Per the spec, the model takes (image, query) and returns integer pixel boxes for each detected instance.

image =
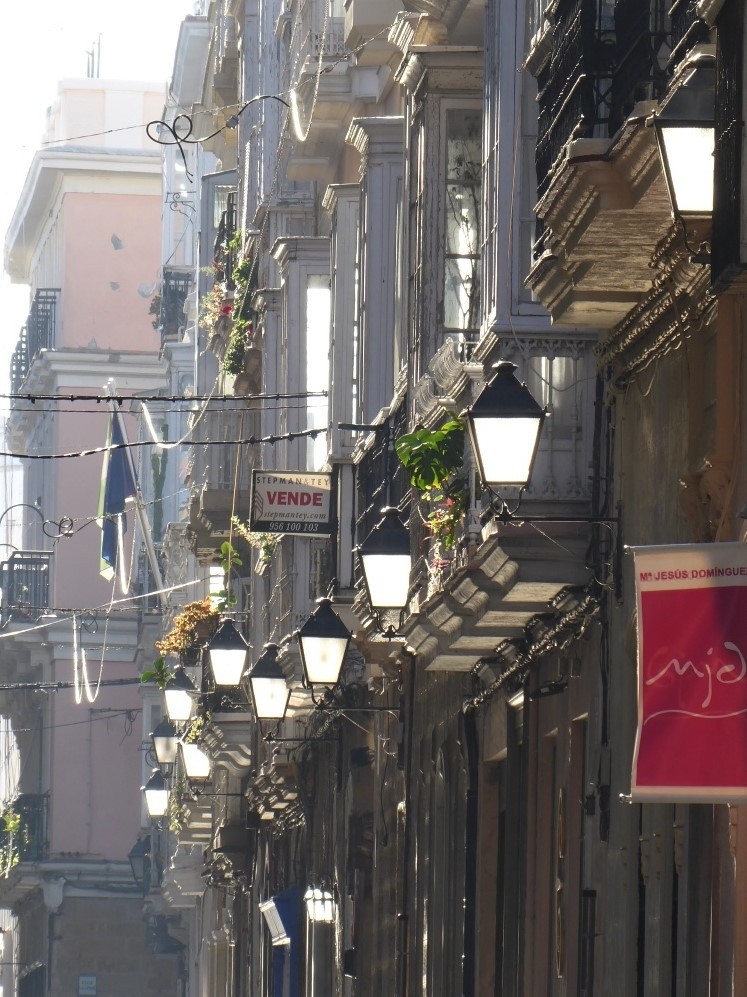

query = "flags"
[96,403,140,581]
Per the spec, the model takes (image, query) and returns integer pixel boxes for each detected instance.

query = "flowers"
[156,596,220,657]
[395,411,472,573]
[196,257,257,375]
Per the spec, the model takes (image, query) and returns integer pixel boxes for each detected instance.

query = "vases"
[180,621,210,667]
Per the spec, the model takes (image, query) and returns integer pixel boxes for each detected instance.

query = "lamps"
[645,54,715,269]
[303,881,339,924]
[243,642,340,742]
[459,362,624,522]
[356,507,411,637]
[179,726,245,796]
[126,834,150,895]
[159,665,197,722]
[296,598,401,711]
[140,715,179,816]
[207,618,252,705]
